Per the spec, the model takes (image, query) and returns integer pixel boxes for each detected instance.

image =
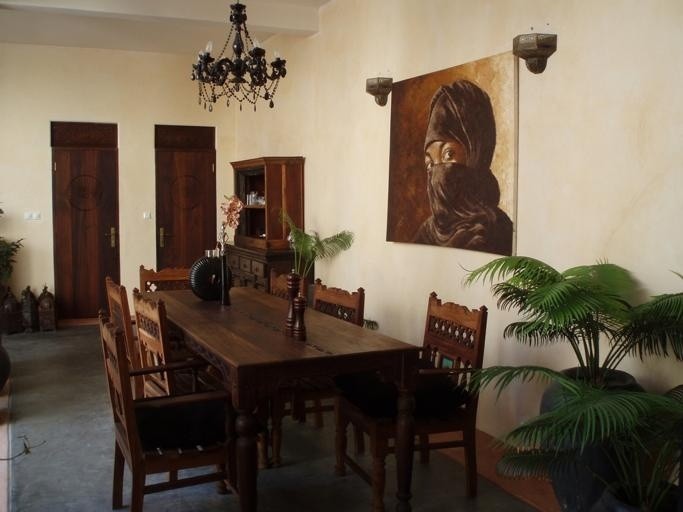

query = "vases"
[219,224,229,305]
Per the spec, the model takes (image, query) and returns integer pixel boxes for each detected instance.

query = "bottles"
[284,273,306,335]
[189,249,232,301]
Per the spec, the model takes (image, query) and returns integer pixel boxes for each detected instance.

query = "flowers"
[219,193,244,231]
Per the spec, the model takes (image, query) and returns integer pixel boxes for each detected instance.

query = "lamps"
[366,78,393,106]
[190,0,286,111]
[513,33,557,73]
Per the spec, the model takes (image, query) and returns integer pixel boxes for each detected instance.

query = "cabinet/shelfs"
[226,157,315,297]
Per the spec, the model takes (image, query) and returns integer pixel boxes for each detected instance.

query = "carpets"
[1,325,541,511]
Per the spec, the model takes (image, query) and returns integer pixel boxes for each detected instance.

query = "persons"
[413,78,511,257]
